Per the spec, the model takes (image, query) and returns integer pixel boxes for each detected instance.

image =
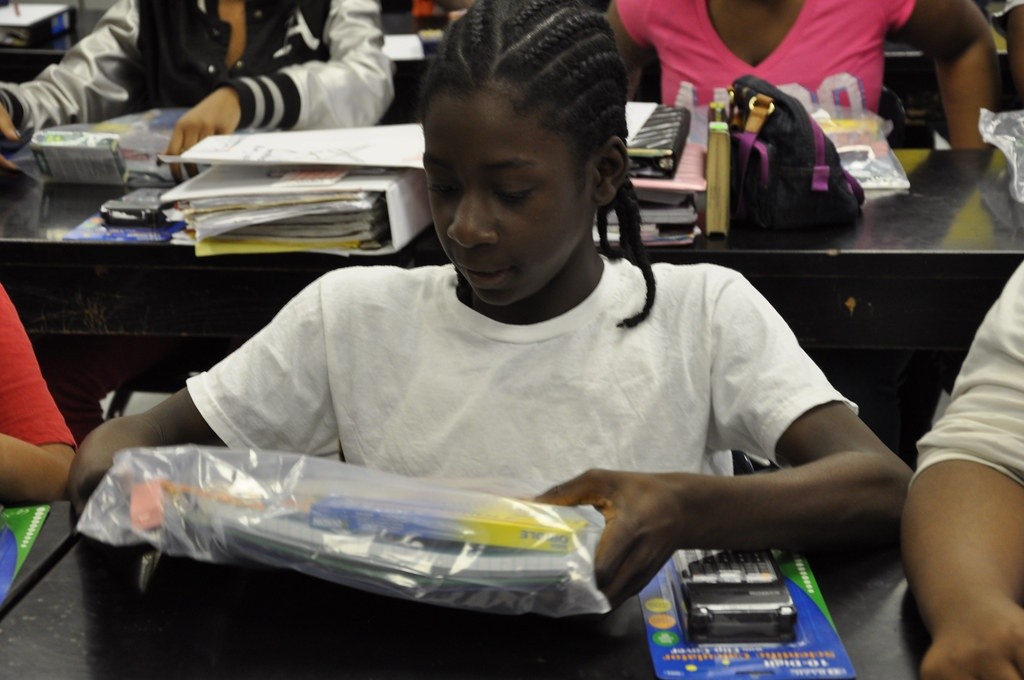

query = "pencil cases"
[624,102,691,181]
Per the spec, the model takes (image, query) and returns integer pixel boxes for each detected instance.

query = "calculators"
[674,547,799,645]
[98,185,170,226]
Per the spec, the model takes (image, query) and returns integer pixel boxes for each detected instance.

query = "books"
[593,189,703,246]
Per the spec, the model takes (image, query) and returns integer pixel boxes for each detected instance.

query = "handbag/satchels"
[710,74,864,240]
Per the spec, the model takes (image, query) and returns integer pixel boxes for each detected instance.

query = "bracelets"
[79,468,108,502]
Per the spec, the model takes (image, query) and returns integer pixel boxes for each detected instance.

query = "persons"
[591,0,998,158]
[0,283,77,504]
[1,0,397,180]
[993,1,1024,106]
[902,262,1023,680]
[70,1,917,614]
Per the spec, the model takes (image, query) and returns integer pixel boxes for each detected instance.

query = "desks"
[0,16,1024,680]
